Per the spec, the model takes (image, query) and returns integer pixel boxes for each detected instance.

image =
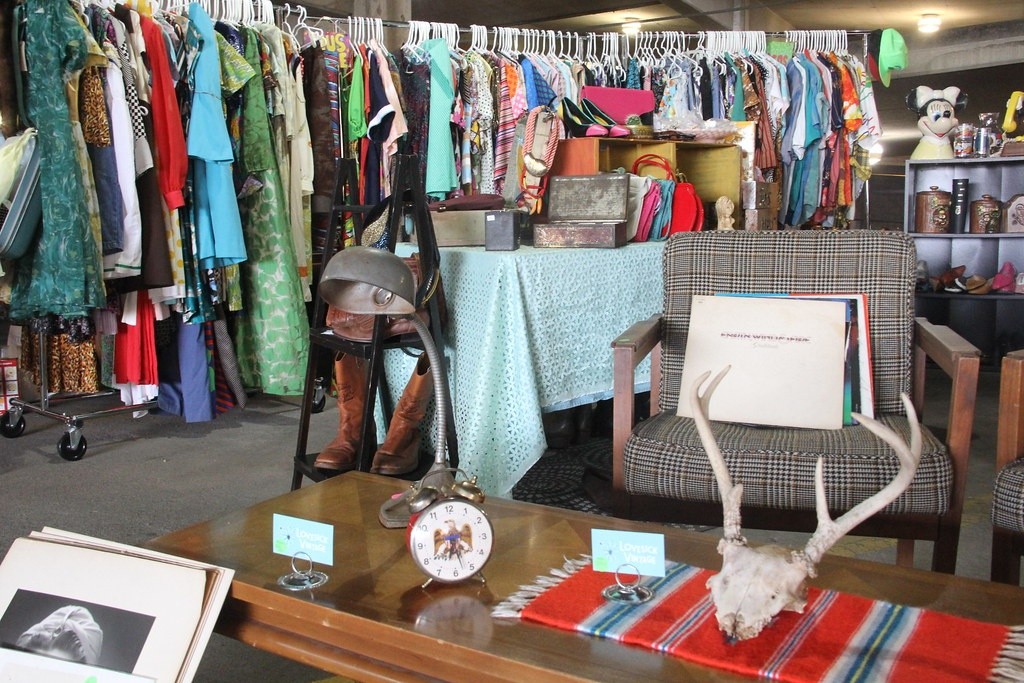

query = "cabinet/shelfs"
[901,156,1023,374]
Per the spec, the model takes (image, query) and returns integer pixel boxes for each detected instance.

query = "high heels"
[557,97,633,140]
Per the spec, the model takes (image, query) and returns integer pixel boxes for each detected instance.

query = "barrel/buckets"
[914,186,951,233]
[970,195,1003,233]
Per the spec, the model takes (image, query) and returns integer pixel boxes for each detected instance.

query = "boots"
[312,349,378,469]
[372,352,434,474]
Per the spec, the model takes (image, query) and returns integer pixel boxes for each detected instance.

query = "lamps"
[318,245,469,529]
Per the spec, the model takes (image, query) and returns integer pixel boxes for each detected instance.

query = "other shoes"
[919,261,1023,296]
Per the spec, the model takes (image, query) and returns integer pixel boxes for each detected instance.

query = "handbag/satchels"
[619,153,706,243]
[327,249,448,342]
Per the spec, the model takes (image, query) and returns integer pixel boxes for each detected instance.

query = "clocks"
[403,466,494,589]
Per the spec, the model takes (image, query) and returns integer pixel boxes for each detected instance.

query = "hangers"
[78,0,881,86]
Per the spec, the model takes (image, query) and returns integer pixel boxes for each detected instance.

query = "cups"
[973,126,991,158]
[951,178,969,233]
[953,122,977,158]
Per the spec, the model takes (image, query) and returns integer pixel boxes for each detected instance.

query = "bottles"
[974,112,1005,153]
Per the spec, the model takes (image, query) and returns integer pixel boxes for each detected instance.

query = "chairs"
[608,228,984,579]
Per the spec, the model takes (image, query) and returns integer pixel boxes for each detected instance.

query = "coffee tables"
[128,466,1023,683]
